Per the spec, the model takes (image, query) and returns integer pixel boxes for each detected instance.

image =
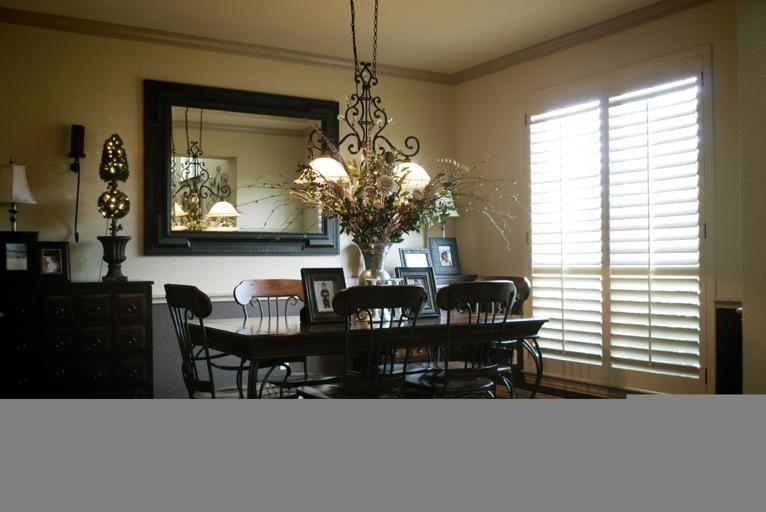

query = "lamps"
[64,123,87,243]
[294,0,432,203]
[169,106,245,232]
[95,132,132,282]
[1,156,39,231]
[427,183,462,237]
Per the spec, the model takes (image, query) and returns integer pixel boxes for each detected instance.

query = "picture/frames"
[426,234,464,276]
[396,246,434,279]
[39,239,72,281]
[300,266,354,323]
[0,229,39,276]
[392,264,441,319]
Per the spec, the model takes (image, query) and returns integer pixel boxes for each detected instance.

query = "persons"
[412,278,425,289]
[45,254,62,274]
[320,283,331,309]
[440,249,452,266]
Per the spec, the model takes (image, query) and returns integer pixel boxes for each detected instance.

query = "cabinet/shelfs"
[1,271,156,399]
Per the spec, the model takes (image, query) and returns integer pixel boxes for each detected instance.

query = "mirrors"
[140,76,344,257]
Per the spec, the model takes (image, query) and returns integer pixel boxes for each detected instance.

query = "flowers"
[236,119,532,252]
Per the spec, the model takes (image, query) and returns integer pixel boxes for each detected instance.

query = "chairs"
[163,283,301,399]
[473,273,532,390]
[234,277,339,388]
[400,280,518,399]
[294,284,428,402]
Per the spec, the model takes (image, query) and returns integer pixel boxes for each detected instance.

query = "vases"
[348,236,399,321]
